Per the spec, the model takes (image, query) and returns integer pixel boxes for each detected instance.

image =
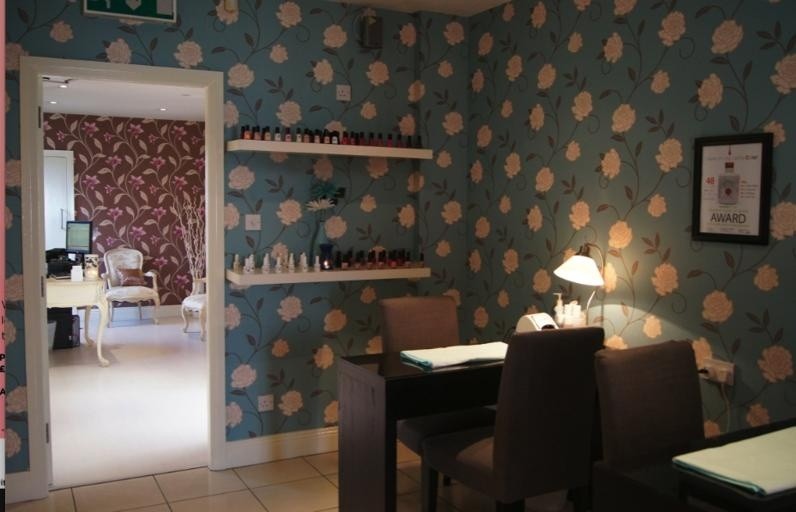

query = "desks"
[46,277,110,367]
[667,417,796,510]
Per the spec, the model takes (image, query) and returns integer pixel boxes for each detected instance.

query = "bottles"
[240,124,424,152]
[234,243,422,273]
[71,254,98,280]
[563,303,587,330]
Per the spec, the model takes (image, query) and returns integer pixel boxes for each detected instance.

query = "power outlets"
[335,84,352,102]
[255,395,275,414]
[700,358,734,387]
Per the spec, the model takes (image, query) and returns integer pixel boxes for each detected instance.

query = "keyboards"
[49,268,71,280]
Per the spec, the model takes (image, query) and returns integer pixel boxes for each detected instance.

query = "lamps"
[553,243,605,326]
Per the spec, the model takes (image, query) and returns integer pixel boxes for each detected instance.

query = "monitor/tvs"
[65,221,93,262]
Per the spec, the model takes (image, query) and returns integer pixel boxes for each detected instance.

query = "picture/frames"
[692,133,774,247]
[82,0,177,24]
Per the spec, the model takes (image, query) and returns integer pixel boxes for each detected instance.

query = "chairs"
[100,249,161,329]
[594,340,705,511]
[181,276,207,343]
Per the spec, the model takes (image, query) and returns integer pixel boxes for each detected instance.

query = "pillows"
[115,268,144,286]
